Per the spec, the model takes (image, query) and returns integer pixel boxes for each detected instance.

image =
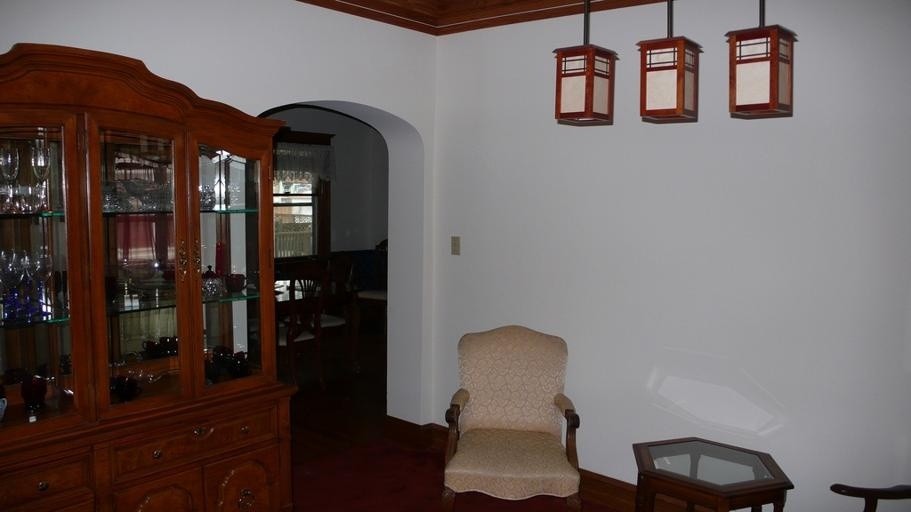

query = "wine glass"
[0,147,56,319]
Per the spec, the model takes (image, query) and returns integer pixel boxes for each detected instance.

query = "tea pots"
[201,264,222,299]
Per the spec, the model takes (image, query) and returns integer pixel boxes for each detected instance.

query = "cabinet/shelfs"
[85,109,276,420]
[98,382,299,512]
[0,421,106,512]
[0,107,97,450]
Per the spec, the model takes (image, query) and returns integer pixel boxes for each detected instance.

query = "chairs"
[441,324,585,511]
[829,484,911,512]
[275,253,352,394]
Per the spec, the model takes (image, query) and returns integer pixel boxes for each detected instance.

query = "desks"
[353,289,387,387]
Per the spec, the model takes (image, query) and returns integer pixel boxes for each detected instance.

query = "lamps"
[552,0,620,126]
[724,0,799,119]
[635,0,703,121]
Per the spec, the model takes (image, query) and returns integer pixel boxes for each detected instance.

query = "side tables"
[632,435,795,512]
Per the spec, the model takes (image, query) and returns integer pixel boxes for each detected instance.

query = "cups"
[141,335,176,357]
[20,377,47,413]
[226,274,248,292]
